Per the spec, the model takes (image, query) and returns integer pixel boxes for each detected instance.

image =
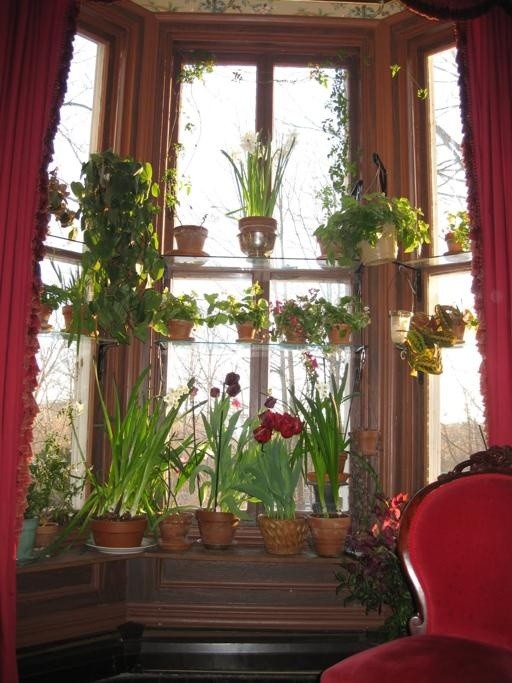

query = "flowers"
[221,128,299,216]
[161,44,214,225]
[332,490,419,639]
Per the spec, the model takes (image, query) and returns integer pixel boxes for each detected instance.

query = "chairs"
[320,444,512,683]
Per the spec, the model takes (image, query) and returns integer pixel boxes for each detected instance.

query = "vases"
[173,225,209,251]
[236,216,279,257]
[348,430,380,456]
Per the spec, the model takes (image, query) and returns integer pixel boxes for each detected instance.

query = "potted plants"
[314,190,471,266]
